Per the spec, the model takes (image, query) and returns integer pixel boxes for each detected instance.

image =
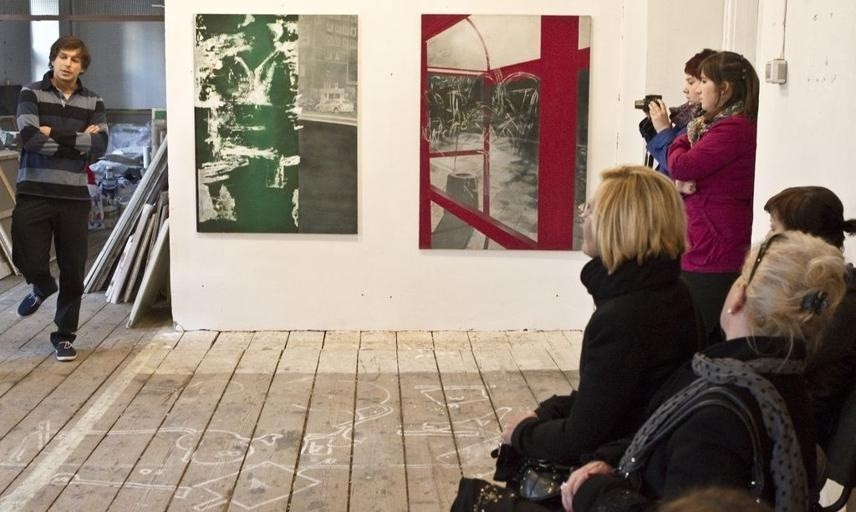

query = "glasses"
[749,233,787,283]
[577,203,593,219]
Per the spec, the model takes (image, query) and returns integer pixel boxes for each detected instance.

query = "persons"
[666,52,760,343]
[763,185,855,512]
[448,228,850,511]
[645,49,721,177]
[9,35,110,362]
[499,163,706,467]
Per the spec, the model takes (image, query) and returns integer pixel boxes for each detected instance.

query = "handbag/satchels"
[520,458,577,501]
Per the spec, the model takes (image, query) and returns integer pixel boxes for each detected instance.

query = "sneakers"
[16,288,58,318]
[54,340,77,361]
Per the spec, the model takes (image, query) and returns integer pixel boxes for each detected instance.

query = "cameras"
[635,94,663,114]
